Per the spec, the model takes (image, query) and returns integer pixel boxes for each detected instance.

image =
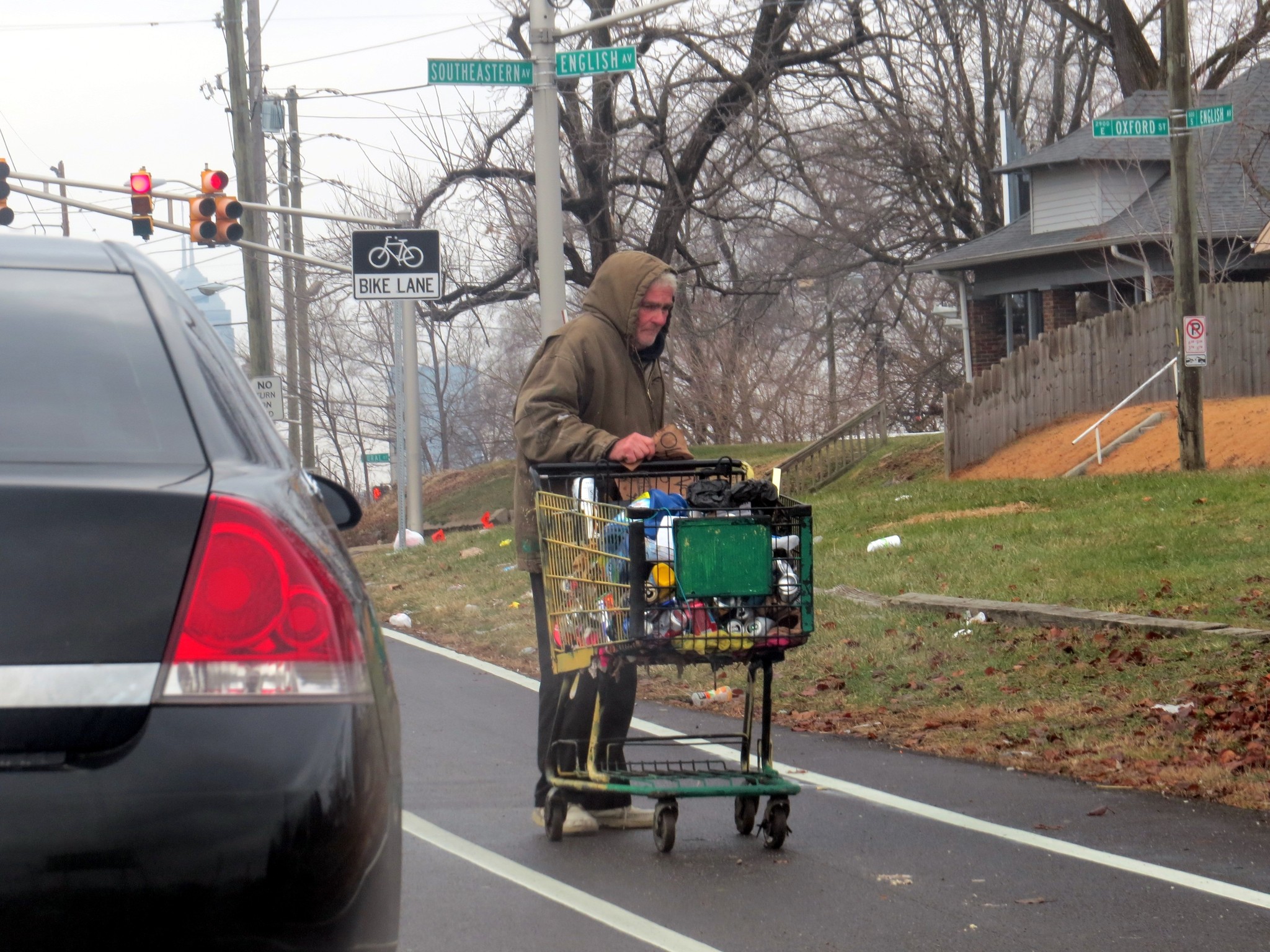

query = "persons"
[512,251,677,832]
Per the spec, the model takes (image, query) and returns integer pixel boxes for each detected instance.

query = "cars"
[0,230,408,952]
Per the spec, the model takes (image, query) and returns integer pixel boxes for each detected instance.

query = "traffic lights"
[0,158,15,227]
[215,195,244,244]
[201,170,229,194]
[129,171,154,236]
[189,195,218,244]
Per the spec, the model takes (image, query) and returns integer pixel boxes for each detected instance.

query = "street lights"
[196,281,300,464]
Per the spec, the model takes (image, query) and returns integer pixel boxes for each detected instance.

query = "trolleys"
[527,457,819,854]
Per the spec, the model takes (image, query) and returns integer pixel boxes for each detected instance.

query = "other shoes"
[532,802,598,833]
[587,804,656,830]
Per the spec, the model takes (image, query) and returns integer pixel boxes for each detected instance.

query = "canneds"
[571,476,601,540]
[552,555,801,649]
